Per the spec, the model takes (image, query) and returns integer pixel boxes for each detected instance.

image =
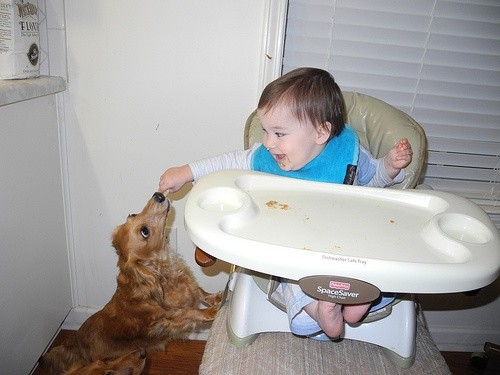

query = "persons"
[160,67,414,339]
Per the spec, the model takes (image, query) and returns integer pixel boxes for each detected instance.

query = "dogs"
[38,192,225,375]
[61,346,147,375]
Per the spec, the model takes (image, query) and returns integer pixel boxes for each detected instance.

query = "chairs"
[193,88,456,375]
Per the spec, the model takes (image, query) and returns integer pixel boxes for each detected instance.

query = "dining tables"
[181,167,500,369]
